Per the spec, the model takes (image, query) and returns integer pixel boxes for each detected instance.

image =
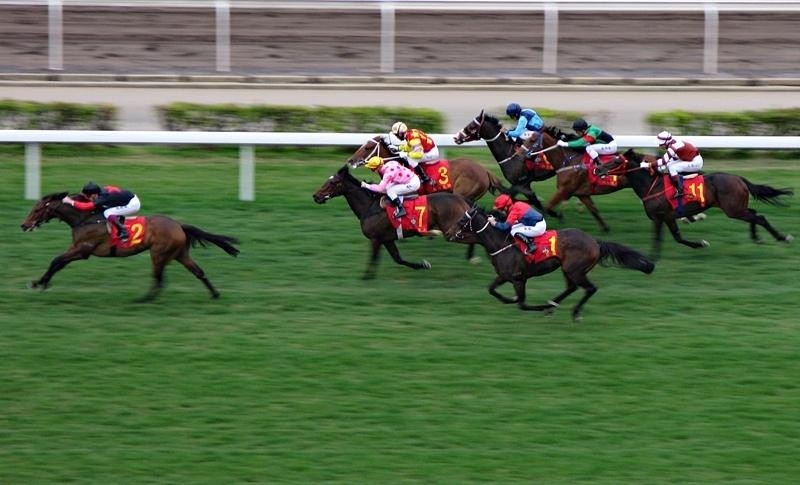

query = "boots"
[113,218,129,239]
[414,164,431,184]
[670,174,684,199]
[593,156,602,166]
[514,232,537,253]
[392,197,407,218]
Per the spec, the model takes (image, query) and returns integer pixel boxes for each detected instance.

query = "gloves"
[639,161,649,168]
[360,181,371,189]
[388,144,399,150]
[396,151,408,158]
[61,196,74,206]
[557,139,568,147]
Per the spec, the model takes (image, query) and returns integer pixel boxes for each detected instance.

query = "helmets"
[570,118,588,130]
[364,156,383,169]
[391,122,408,135]
[656,131,673,144]
[82,182,101,195]
[506,102,521,119]
[494,193,512,208]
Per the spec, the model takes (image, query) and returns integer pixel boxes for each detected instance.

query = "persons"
[63,182,141,240]
[557,119,617,166]
[361,156,421,218]
[388,121,439,184]
[487,194,546,256]
[500,103,544,154]
[640,130,703,199]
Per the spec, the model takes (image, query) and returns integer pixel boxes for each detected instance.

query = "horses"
[445,203,655,323]
[346,136,509,202]
[515,124,707,233]
[20,191,241,304]
[312,163,483,280]
[452,108,565,221]
[595,147,795,259]
